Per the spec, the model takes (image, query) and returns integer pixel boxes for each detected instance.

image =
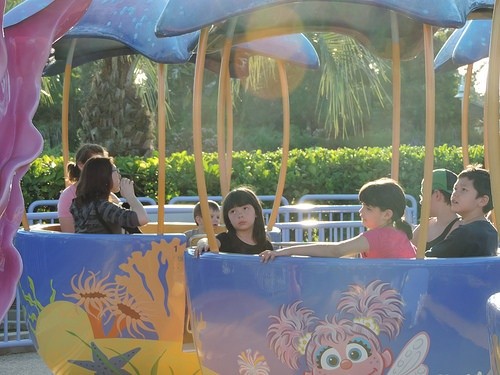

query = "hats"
[421,169,458,195]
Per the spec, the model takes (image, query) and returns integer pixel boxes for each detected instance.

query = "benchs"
[13,222,500,375]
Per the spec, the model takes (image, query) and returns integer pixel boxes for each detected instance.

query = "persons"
[410,168,500,258]
[184,188,273,258]
[259,179,418,264]
[58,143,149,234]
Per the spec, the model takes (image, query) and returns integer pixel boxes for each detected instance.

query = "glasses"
[112,167,121,173]
